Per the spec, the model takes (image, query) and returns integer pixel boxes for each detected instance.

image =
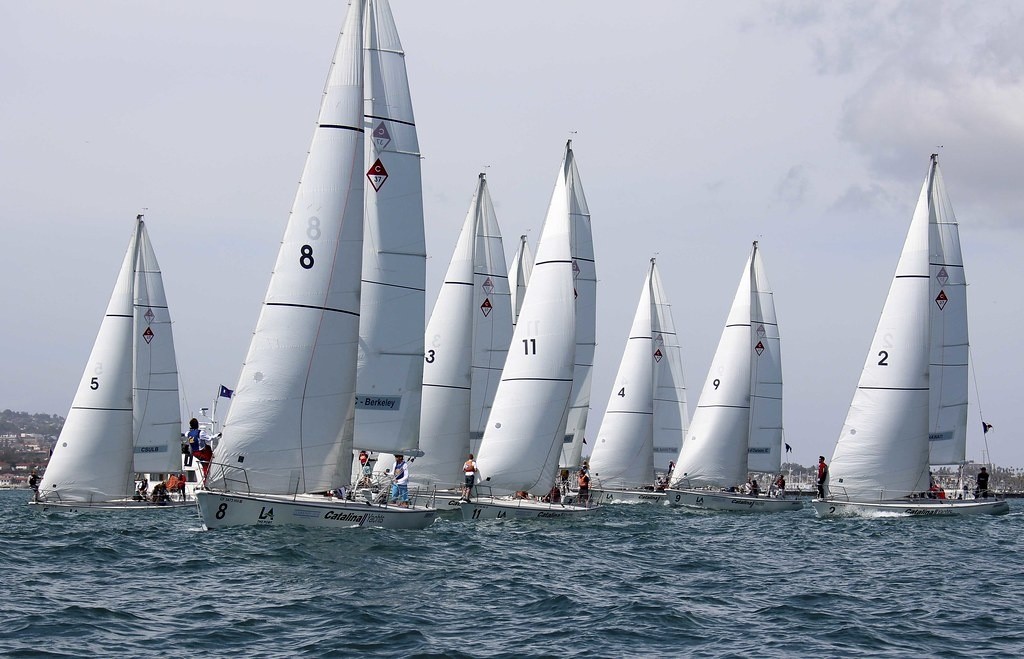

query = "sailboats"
[808,148,1014,517]
[663,238,806,512]
[570,256,693,503]
[138,1,602,529]
[25,208,201,512]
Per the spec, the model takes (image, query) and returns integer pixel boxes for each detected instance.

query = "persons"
[657,460,674,485]
[185,419,222,491]
[775,475,785,490]
[816,456,828,499]
[577,470,589,505]
[152,473,185,501]
[582,461,589,473]
[727,487,735,491]
[359,450,371,477]
[385,455,409,506]
[977,468,989,490]
[749,479,758,495]
[560,470,570,494]
[928,473,935,485]
[461,454,477,502]
[137,478,148,501]
[28,471,44,501]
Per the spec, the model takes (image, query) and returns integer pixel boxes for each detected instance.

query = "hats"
[981,467,986,470]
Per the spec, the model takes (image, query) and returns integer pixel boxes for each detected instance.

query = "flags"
[220,385,233,398]
